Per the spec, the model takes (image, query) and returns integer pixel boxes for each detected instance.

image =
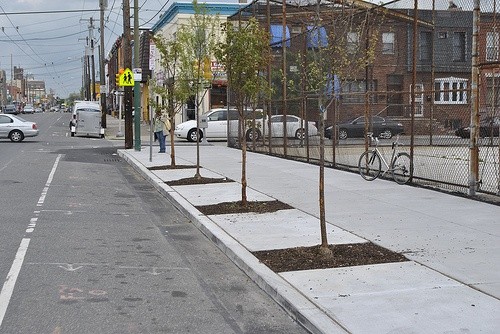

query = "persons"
[153,107,170,154]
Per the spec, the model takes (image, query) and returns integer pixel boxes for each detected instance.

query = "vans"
[69,100,105,138]
[173,108,270,142]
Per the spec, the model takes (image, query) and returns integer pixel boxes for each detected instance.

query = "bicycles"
[358,134,414,185]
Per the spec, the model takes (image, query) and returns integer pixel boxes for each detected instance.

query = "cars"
[455,117,500,137]
[267,115,317,140]
[0,114,39,142]
[3,104,43,114]
[325,115,404,139]
